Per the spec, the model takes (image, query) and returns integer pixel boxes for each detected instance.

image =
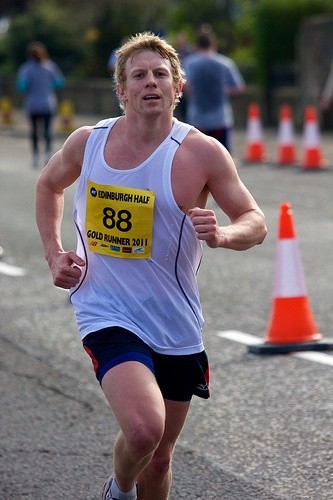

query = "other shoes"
[102,474,138,500]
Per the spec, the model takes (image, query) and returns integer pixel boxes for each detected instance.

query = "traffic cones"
[236,102,266,167]
[297,107,331,171]
[246,203,333,361]
[269,103,297,170]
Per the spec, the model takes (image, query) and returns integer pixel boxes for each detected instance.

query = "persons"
[15,43,63,169]
[35,35,269,500]
[108,23,244,156]
